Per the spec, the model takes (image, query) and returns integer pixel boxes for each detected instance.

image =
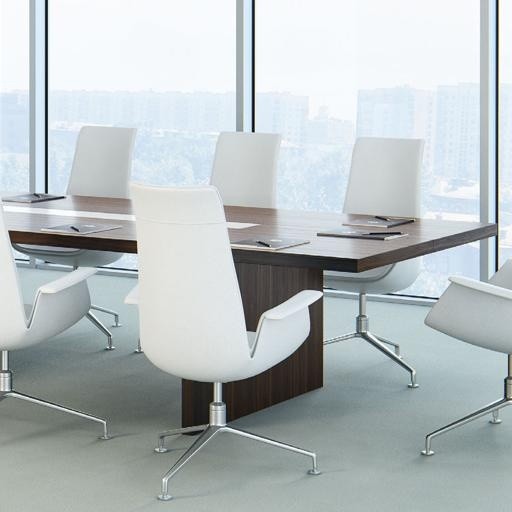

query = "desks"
[1,193,497,435]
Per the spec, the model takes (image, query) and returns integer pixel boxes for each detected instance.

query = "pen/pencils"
[257,241,270,247]
[34,193,42,198]
[362,232,403,234]
[70,226,79,232]
[375,216,392,222]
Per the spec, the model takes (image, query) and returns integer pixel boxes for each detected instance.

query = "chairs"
[419,261,511,457]
[1,210,112,441]
[207,131,281,211]
[324,136,426,389]
[124,182,324,502]
[12,126,138,350]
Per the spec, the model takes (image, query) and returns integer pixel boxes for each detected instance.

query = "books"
[42,222,122,234]
[341,218,415,228]
[1,194,66,202]
[316,227,408,239]
[229,237,309,250]
